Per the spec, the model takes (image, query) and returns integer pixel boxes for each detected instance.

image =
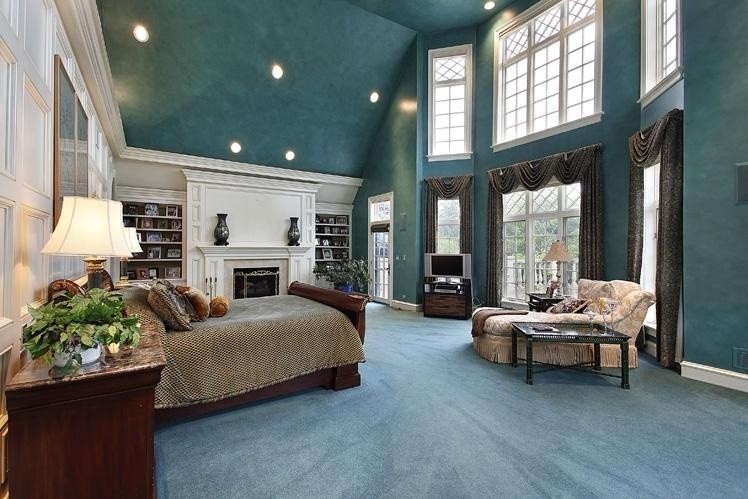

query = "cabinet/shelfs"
[6,322,155,499]
[423,281,473,320]
[314,212,352,288]
[116,204,186,286]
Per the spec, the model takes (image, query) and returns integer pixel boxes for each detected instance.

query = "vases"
[287,217,300,246]
[213,213,232,246]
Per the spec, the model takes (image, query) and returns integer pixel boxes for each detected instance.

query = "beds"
[49,271,368,429]
[471,280,657,368]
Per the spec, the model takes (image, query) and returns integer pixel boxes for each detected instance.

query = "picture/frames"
[54,54,89,232]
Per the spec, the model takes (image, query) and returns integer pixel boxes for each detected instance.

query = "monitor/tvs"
[423,252,472,284]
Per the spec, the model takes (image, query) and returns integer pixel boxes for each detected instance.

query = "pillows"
[210,296,228,318]
[150,278,193,331]
[183,284,208,324]
[549,296,591,314]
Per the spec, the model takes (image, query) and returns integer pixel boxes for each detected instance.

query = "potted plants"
[23,290,140,374]
[314,257,371,295]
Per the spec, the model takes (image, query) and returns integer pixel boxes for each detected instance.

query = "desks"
[526,293,562,313]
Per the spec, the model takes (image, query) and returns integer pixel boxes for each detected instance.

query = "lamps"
[541,241,574,298]
[113,227,143,285]
[38,195,133,292]
